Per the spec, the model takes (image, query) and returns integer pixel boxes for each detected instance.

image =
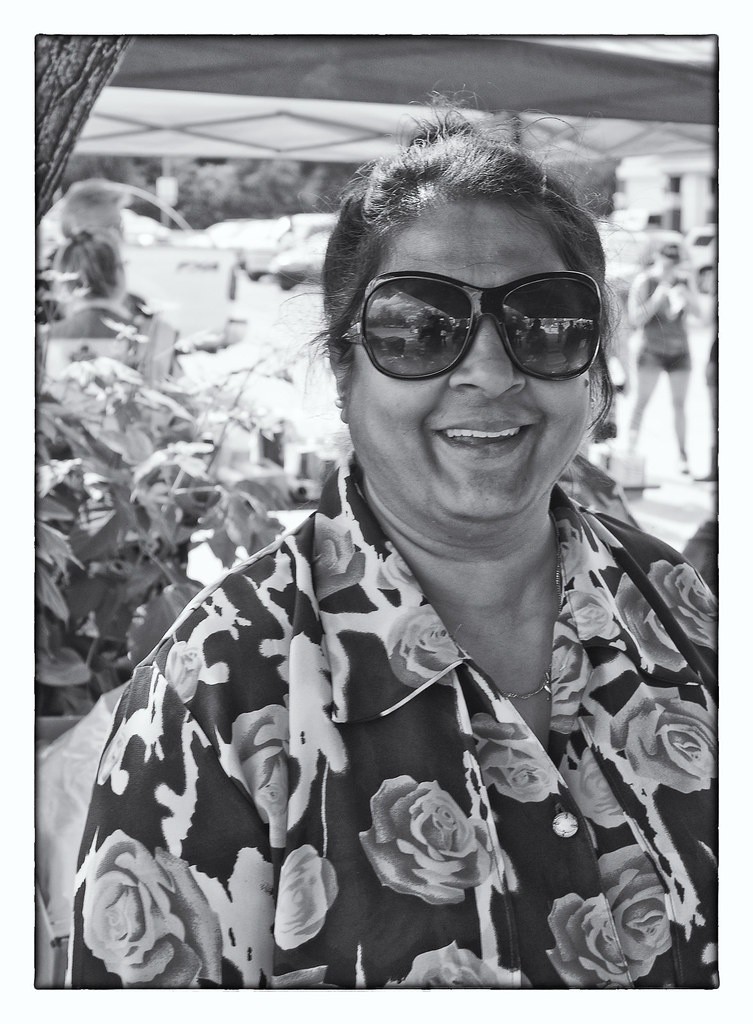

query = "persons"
[695,239,718,483]
[69,103,719,991]
[622,241,693,475]
[36,188,185,379]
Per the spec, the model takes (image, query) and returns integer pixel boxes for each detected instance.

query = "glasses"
[660,251,680,260]
[341,272,606,384]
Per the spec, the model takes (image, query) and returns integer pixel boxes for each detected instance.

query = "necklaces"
[449,510,562,702]
